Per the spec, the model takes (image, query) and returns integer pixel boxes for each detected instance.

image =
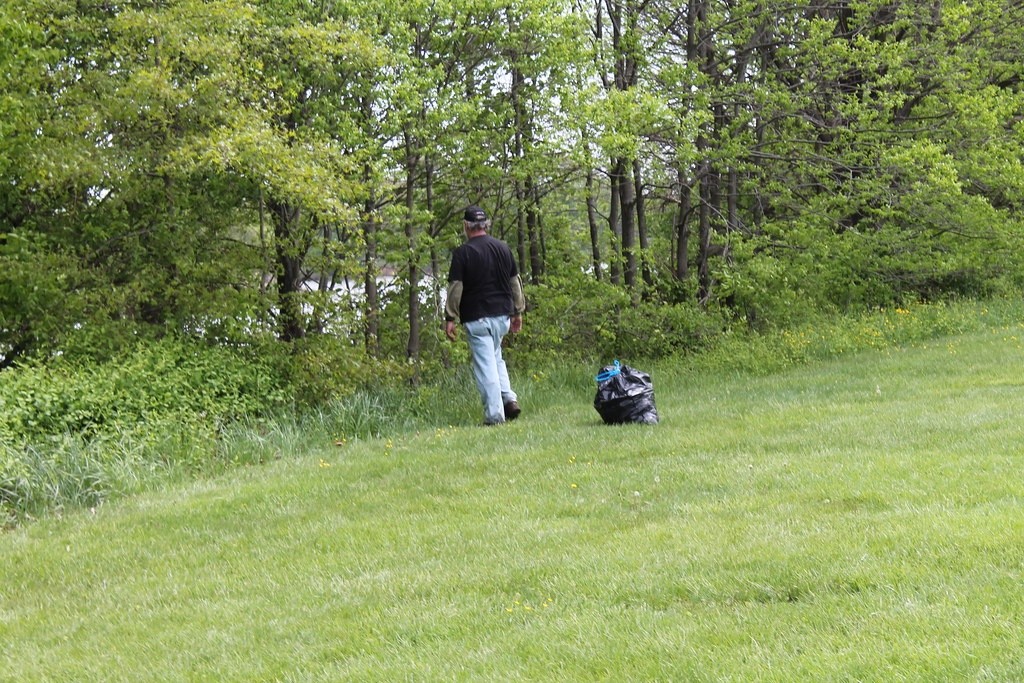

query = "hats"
[464,206,486,222]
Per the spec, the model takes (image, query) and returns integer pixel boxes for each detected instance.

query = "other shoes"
[504,401,521,420]
[483,421,495,426]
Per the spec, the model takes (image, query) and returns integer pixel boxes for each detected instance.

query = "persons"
[445,206,525,426]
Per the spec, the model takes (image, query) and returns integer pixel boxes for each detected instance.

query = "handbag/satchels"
[593,360,659,425]
